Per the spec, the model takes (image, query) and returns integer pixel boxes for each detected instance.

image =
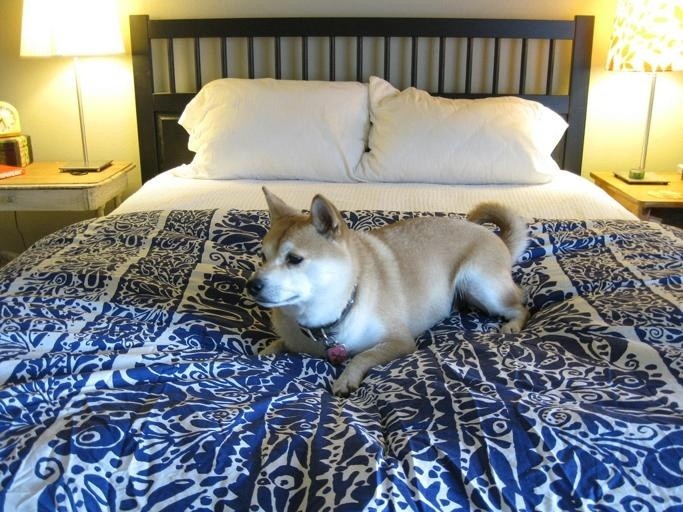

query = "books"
[0,164,23,180]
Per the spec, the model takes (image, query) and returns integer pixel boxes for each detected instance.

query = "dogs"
[245,185,530,399]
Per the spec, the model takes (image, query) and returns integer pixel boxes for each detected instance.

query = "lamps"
[605,0,682,187]
[19,1,126,175]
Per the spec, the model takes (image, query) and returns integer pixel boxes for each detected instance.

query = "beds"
[0,16,683,512]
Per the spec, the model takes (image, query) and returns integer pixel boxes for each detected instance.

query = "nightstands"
[589,168,683,223]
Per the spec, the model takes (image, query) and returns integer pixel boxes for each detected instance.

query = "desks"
[0,164,136,218]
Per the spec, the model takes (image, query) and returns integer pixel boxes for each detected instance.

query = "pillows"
[183,78,370,183]
[353,78,569,184]
